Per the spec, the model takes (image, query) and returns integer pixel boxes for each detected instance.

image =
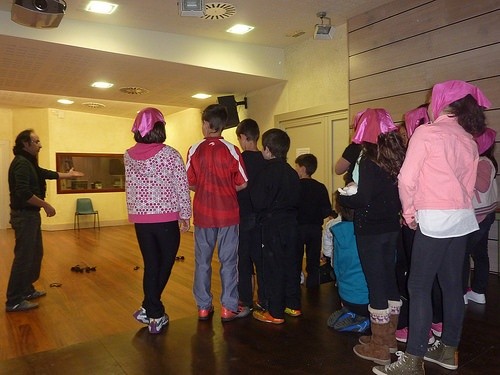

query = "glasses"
[30,140,40,144]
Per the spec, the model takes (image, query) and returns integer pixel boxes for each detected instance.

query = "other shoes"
[6,299,39,311]
[198,304,214,319]
[463,293,468,305]
[221,303,250,320]
[466,287,486,304]
[25,290,46,301]
[326,307,358,327]
[333,315,370,332]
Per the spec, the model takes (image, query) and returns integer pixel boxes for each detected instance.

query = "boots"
[353,304,391,365]
[359,298,403,353]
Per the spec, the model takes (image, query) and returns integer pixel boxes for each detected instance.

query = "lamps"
[178,0,204,16]
[314,12,335,41]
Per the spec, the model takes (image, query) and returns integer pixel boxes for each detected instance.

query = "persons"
[322,177,371,334]
[296,154,331,287]
[236,118,268,310]
[6,129,84,312]
[335,108,406,364]
[186,104,250,320]
[372,79,493,375]
[395,105,442,344]
[252,128,303,324]
[124,107,192,334]
[463,127,500,304]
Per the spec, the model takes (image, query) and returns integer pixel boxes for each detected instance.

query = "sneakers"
[430,321,442,337]
[372,351,425,375]
[423,340,459,369]
[284,307,301,316]
[253,310,284,324]
[148,313,169,333]
[395,327,435,344]
[132,305,149,324]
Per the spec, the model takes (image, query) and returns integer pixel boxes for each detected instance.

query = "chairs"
[74,197,100,229]
